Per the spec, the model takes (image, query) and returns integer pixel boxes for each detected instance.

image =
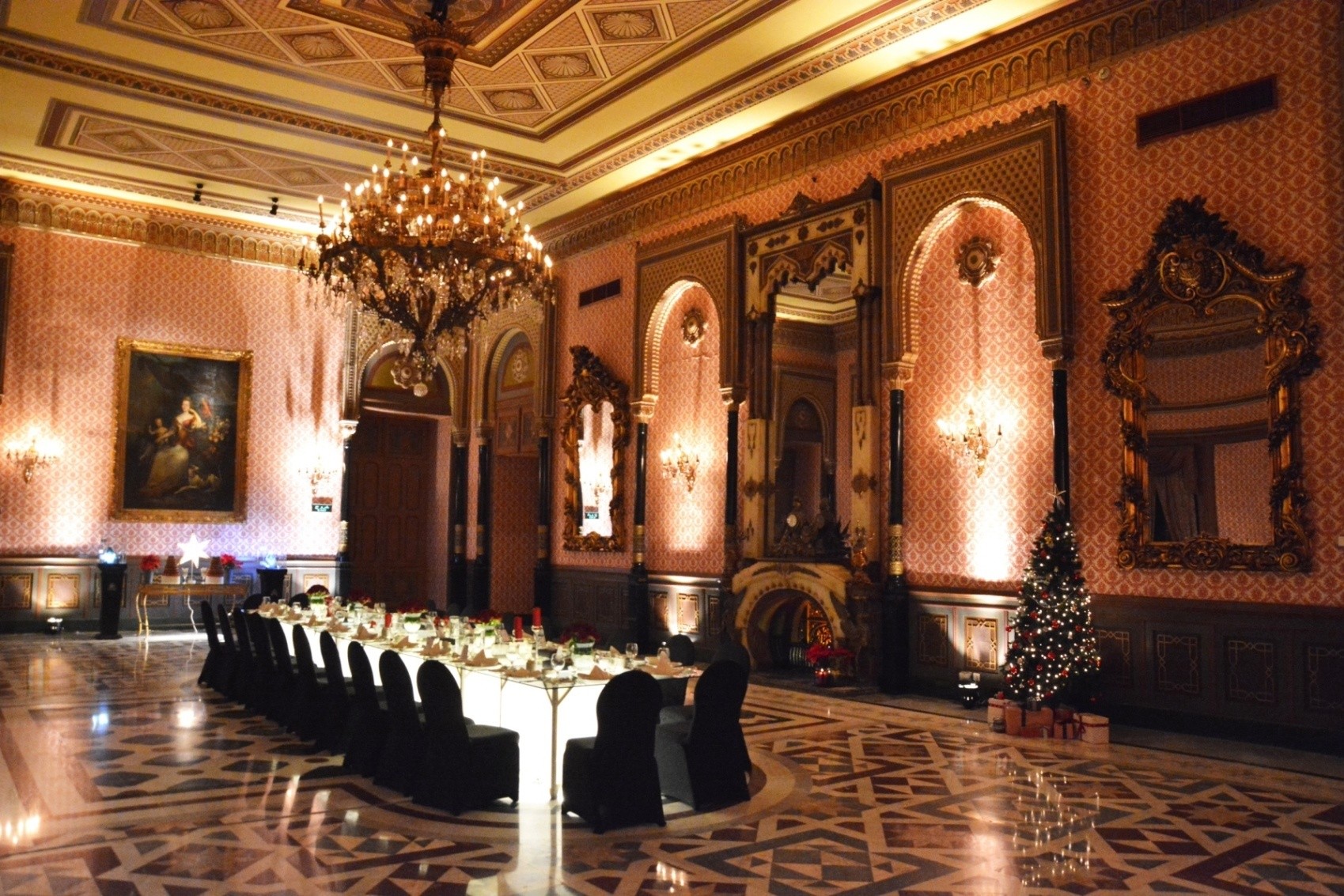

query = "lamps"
[296,1,558,397]
[658,435,702,492]
[5,435,57,484]
[933,404,1010,476]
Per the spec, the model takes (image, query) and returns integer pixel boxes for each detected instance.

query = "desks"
[134,582,248,638]
[253,603,703,799]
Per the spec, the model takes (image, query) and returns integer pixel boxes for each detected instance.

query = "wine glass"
[261,592,669,687]
[200,567,208,585]
[181,567,189,585]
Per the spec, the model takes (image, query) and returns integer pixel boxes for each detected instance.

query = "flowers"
[139,553,161,572]
[221,555,245,569]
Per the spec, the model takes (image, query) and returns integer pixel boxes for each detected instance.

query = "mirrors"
[1099,198,1313,578]
[734,170,888,572]
[557,346,630,552]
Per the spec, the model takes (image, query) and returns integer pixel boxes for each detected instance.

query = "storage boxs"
[987,698,1109,745]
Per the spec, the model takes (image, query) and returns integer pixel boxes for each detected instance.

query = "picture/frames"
[103,332,252,523]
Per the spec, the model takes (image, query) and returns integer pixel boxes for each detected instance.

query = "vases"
[224,568,235,584]
[140,570,154,583]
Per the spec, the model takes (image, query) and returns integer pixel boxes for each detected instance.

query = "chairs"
[192,596,765,833]
